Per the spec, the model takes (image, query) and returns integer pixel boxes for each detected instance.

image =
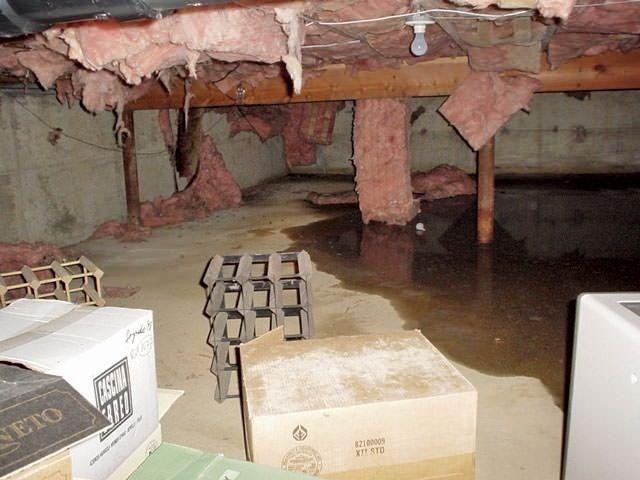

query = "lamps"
[406,21,434,56]
